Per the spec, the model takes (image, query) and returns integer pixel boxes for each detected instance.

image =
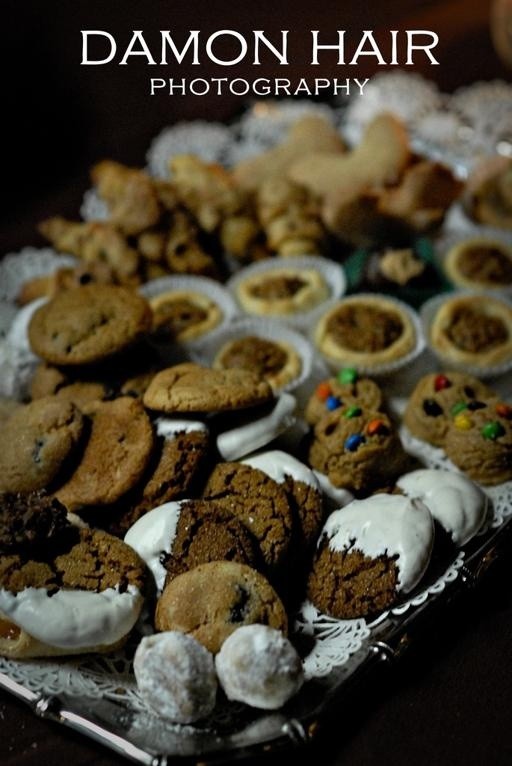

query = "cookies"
[152,561,288,655]
[0,492,143,662]
[0,283,153,511]
[303,367,383,424]
[405,370,492,447]
[308,404,408,492]
[123,499,255,602]
[142,416,208,510]
[445,396,512,485]
[237,449,323,546]
[143,363,271,414]
[195,463,292,564]
[394,467,490,557]
[309,468,355,508]
[306,493,435,617]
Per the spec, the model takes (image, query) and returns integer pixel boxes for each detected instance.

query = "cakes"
[237,268,327,317]
[429,293,510,376]
[447,237,512,294]
[210,335,300,389]
[144,290,221,345]
[316,297,415,367]
